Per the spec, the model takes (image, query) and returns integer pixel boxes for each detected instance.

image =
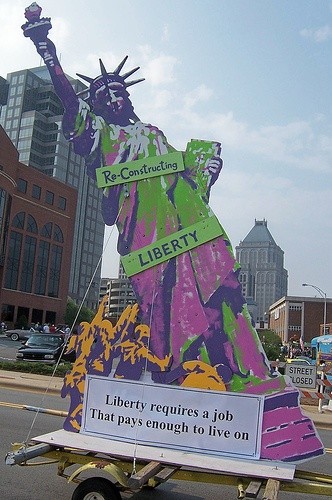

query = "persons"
[35,321,56,334]
[290,334,311,357]
[3,321,8,328]
[63,325,71,334]
[316,360,327,393]
[279,353,286,362]
[280,345,285,352]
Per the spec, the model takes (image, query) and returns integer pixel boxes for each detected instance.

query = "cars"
[5,327,40,342]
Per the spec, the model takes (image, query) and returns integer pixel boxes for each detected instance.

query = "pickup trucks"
[15,333,75,363]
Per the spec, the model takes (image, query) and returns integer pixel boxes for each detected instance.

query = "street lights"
[301,283,327,336]
